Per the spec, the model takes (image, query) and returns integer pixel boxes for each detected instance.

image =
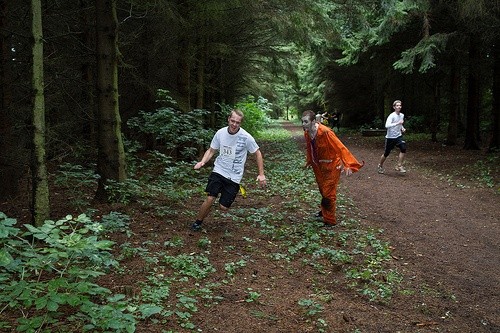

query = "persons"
[191,108,267,232]
[377,99,408,174]
[315,108,354,135]
[297,110,365,230]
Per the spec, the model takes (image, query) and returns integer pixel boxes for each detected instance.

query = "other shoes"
[395,163,407,173]
[191,219,203,231]
[377,163,384,174]
[315,210,323,217]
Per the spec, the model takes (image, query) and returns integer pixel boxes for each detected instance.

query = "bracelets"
[199,162,205,166]
